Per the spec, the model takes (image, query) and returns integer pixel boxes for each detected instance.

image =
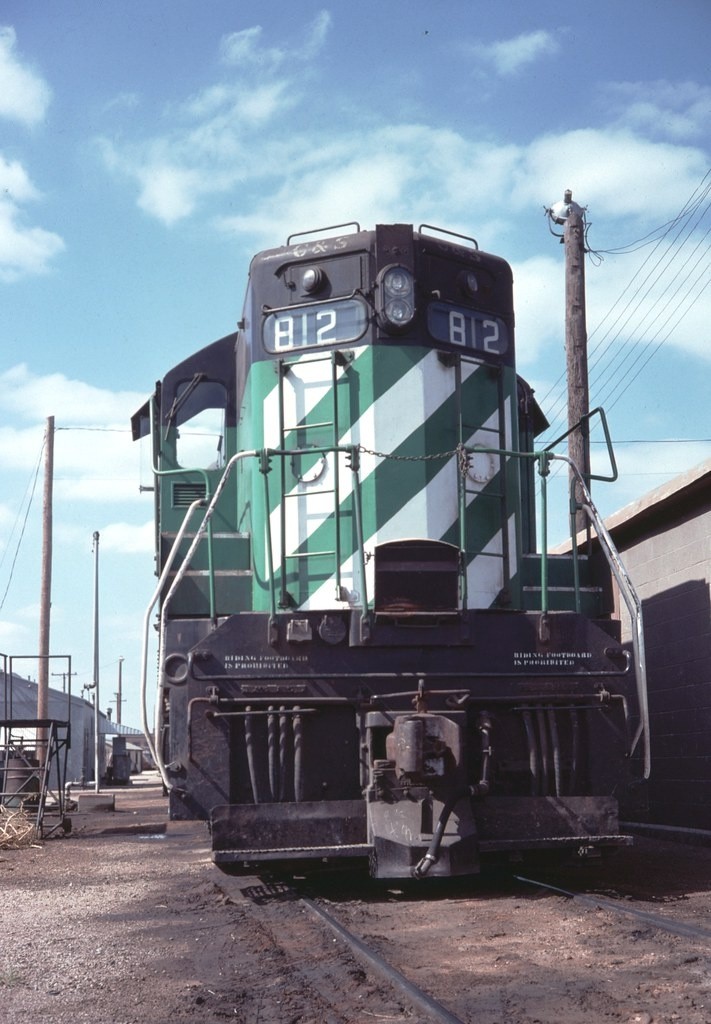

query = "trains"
[131,221,649,879]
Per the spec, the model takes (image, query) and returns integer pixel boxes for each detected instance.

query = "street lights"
[91,532,100,794]
[116,655,124,724]
[544,190,593,534]
